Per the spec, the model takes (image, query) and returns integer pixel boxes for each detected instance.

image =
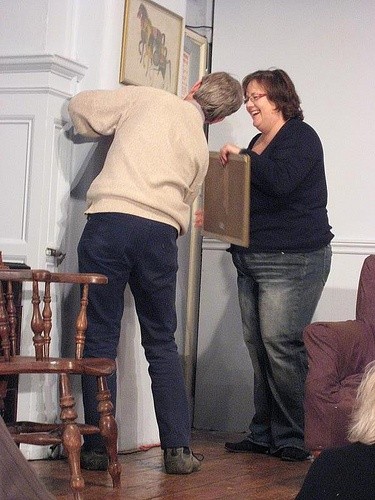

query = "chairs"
[0,252,122,500]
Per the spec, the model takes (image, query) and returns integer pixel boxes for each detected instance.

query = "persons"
[60,73,245,474]
[193,68,335,460]
[294,360,375,500]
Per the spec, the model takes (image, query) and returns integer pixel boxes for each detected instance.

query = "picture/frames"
[182,27,208,96]
[199,150,251,247]
[120,0,184,96]
[68,134,115,200]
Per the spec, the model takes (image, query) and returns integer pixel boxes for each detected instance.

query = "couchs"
[304,256,375,461]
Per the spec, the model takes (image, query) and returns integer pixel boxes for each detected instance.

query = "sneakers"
[278,447,303,463]
[225,440,273,454]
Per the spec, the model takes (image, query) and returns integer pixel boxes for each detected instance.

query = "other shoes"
[80,445,114,473]
[161,448,205,475]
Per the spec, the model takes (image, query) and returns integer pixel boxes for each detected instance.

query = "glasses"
[240,91,273,101]
